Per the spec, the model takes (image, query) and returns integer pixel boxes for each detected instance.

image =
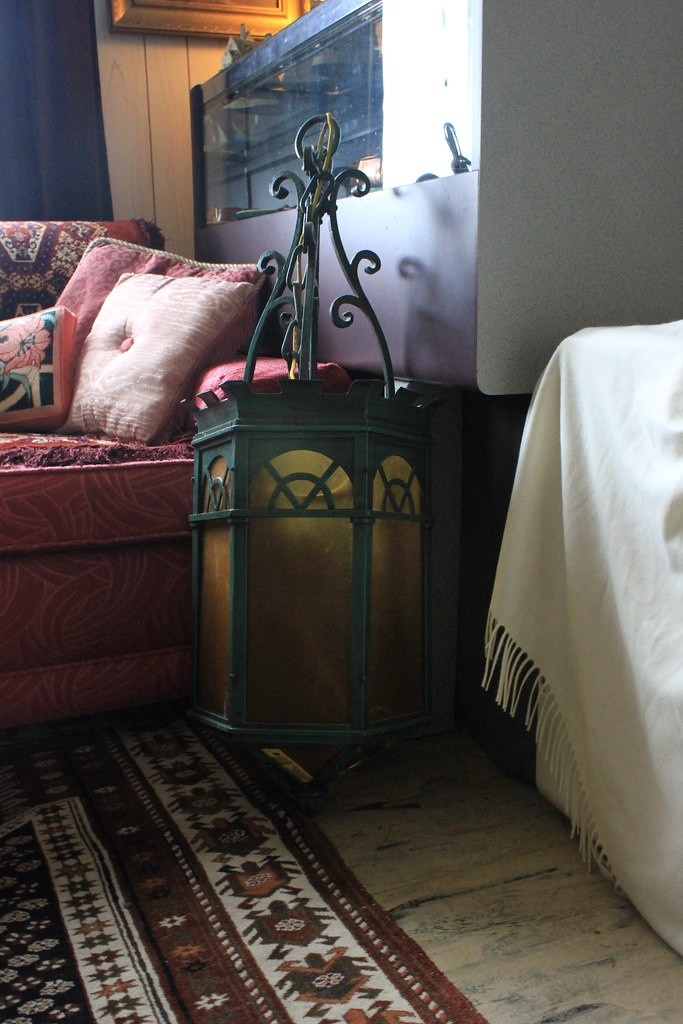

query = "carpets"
[1,692,490,1024]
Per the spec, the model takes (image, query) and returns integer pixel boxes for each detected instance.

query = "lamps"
[173,101,442,793]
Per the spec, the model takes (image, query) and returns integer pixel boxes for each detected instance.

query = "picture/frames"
[107,0,311,43]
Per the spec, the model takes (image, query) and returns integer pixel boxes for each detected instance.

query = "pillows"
[1,306,74,430]
[49,237,269,431]
[59,270,256,451]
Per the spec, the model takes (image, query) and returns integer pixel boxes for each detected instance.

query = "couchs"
[0,212,344,726]
